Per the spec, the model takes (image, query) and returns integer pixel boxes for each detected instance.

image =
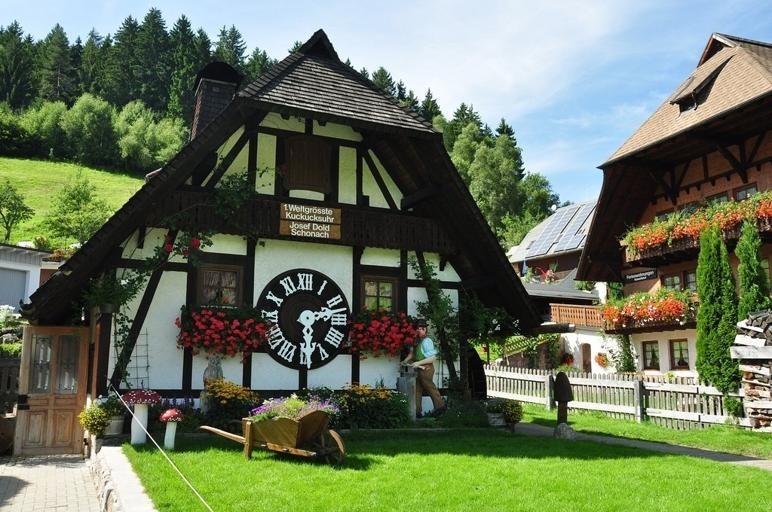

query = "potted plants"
[486,399,523,426]
[99,396,127,435]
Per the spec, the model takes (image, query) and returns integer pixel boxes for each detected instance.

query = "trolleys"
[195,402,345,469]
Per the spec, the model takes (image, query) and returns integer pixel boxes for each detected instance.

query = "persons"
[401,319,447,417]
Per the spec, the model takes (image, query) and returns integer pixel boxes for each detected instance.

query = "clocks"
[257,268,351,370]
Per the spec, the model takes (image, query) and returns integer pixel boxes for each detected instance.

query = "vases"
[242,411,328,448]
[131,404,148,445]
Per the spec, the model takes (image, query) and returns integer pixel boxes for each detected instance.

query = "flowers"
[120,389,162,407]
[250,394,342,424]
